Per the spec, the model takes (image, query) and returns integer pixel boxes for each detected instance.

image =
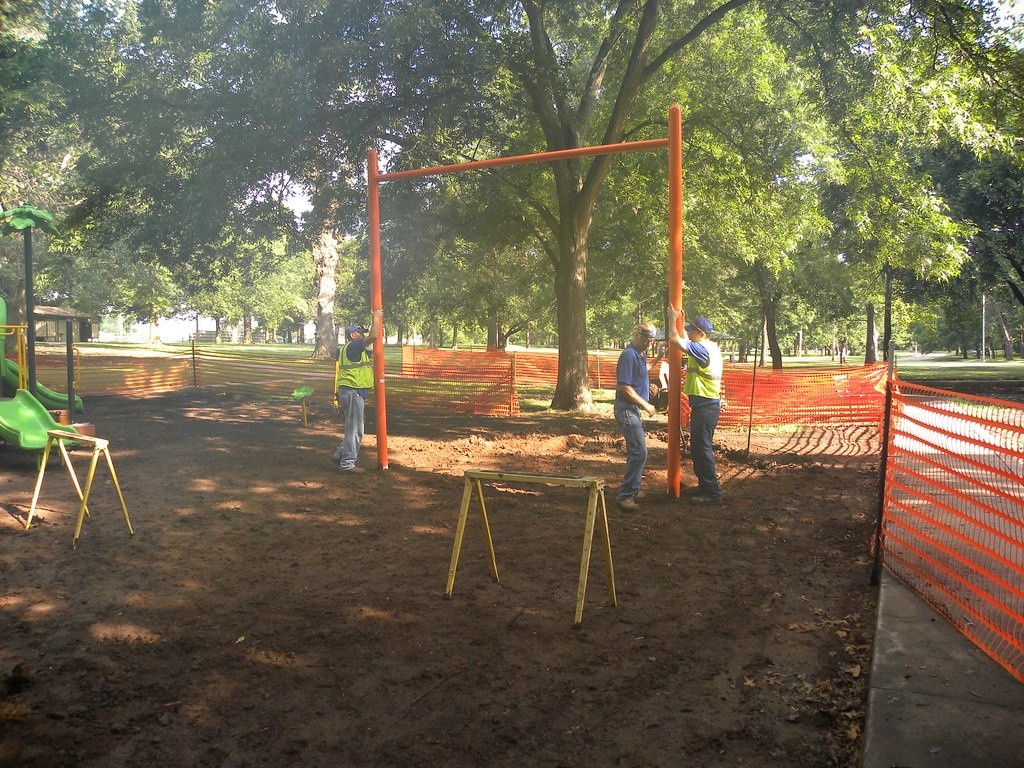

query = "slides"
[0,389,78,450]
[3,357,84,413]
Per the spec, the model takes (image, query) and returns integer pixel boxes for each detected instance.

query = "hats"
[347,324,368,335]
[684,316,714,332]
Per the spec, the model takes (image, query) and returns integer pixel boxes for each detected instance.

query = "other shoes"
[617,490,645,510]
[340,466,366,474]
[689,484,723,505]
[332,458,340,464]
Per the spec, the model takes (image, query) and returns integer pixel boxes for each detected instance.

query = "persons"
[666,300,723,505]
[614,320,657,511]
[334,304,384,474]
